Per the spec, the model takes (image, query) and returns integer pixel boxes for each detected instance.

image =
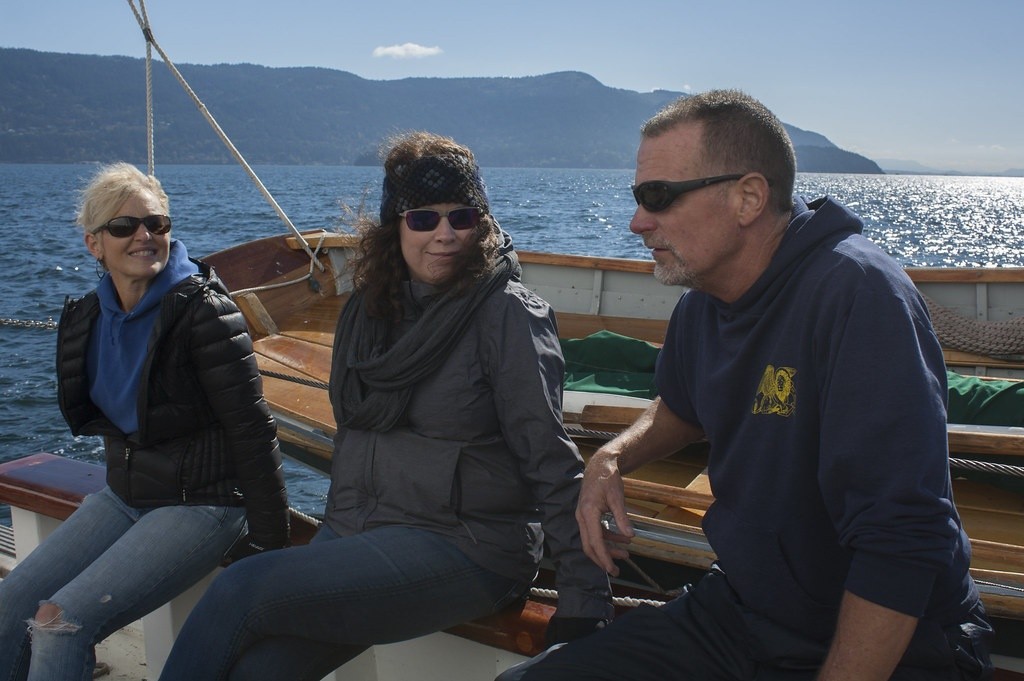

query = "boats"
[2,2,1023,680]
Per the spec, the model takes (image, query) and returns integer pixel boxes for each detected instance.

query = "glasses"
[398,207,482,232]
[631,174,774,212]
[91,215,172,238]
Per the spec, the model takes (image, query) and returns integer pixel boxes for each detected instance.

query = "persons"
[154,127,618,680]
[0,160,291,681]
[487,88,999,681]
